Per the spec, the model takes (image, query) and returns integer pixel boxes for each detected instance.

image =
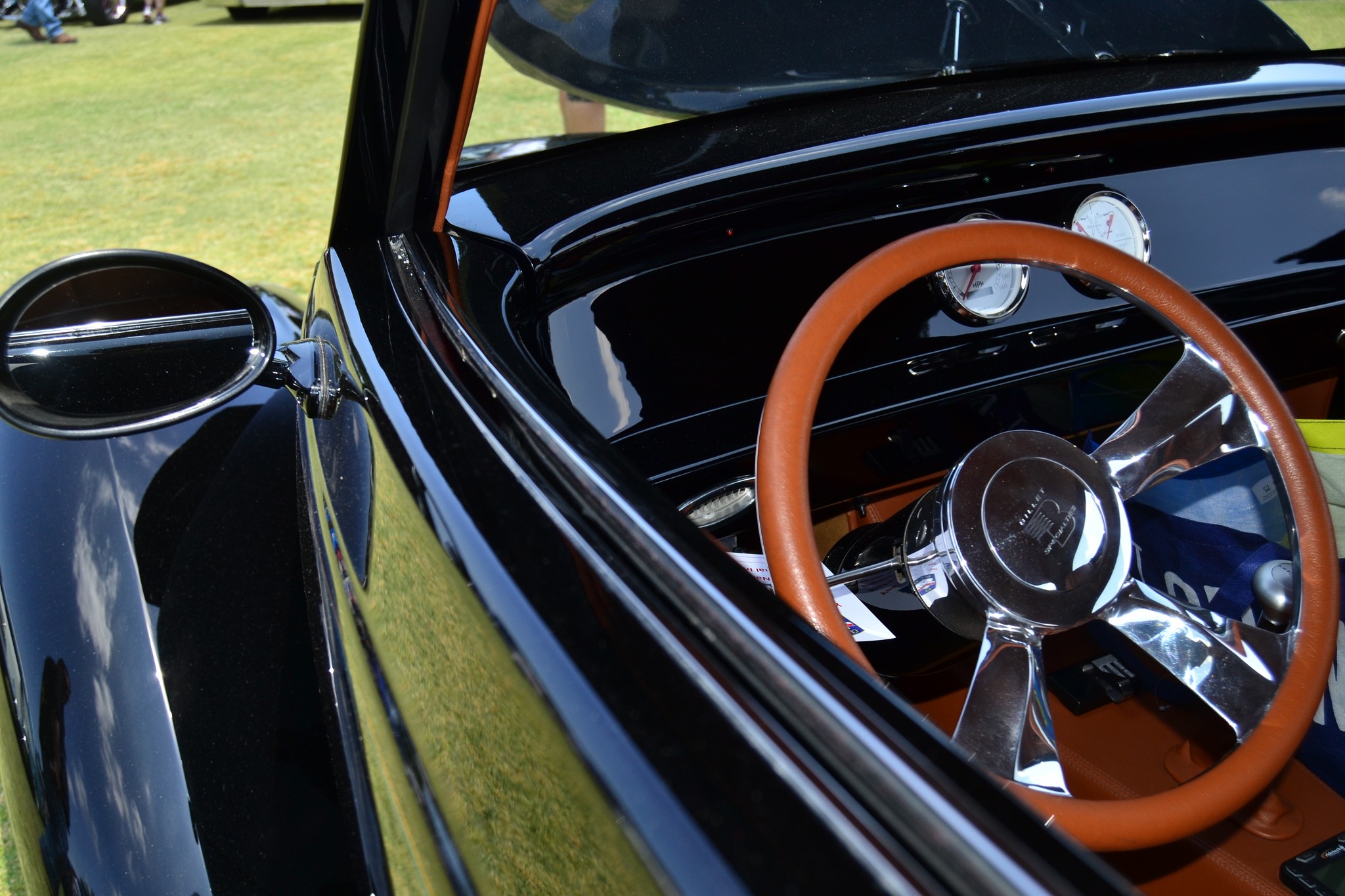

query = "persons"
[17,0,77,42]
[141,0,167,22]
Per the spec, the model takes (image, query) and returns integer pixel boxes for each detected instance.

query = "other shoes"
[51,33,77,43]
[17,19,46,41]
[143,7,153,23]
[155,13,169,25]
